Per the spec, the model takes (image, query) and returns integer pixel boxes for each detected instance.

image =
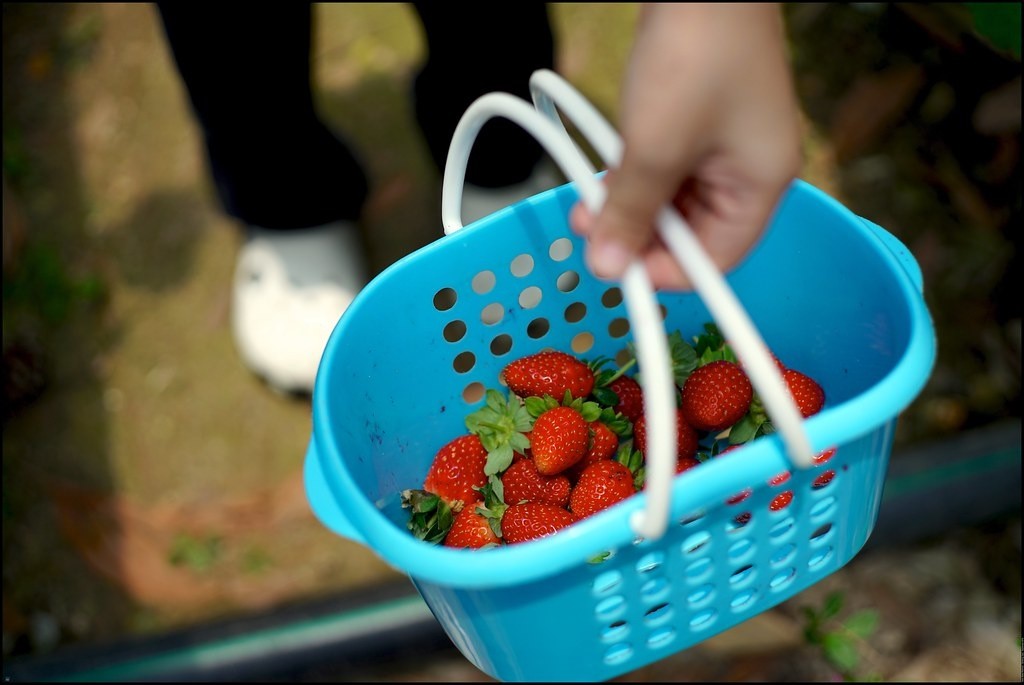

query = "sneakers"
[460,156,563,234]
[229,217,366,395]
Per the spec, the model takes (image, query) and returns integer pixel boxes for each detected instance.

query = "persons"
[146,2,808,404]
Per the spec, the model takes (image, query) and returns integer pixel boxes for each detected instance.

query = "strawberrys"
[399,323,835,549]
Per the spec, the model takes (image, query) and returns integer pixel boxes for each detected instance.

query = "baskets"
[296,61,940,684]
